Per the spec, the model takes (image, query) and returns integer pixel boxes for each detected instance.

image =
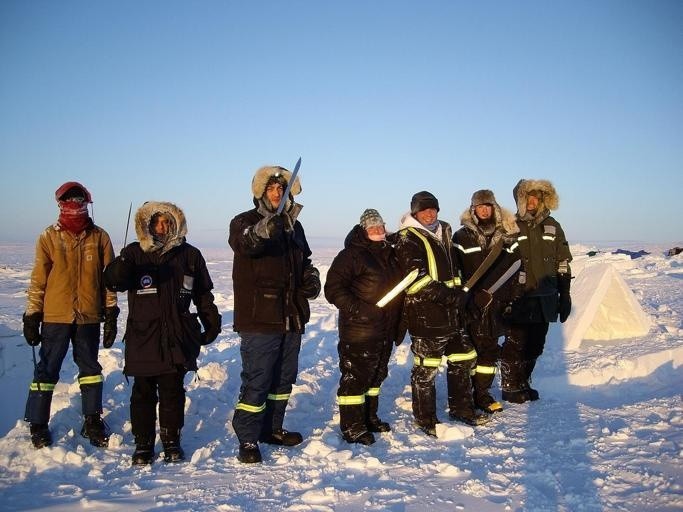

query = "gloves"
[201,330,218,345]
[103,305,120,349]
[559,293,571,323]
[22,311,42,346]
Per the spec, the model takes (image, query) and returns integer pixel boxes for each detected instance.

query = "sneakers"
[29,421,52,448]
[340,387,539,444]
[81,416,109,447]
[132,435,155,467]
[261,429,304,446]
[239,441,262,463]
[160,428,185,463]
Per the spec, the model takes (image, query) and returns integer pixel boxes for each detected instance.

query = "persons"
[453,190,524,413]
[393,190,494,439]
[103,201,221,468]
[323,206,406,448]
[20,180,118,452]
[227,165,321,463]
[503,179,574,406]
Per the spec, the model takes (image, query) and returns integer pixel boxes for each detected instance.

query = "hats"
[411,191,439,218]
[470,190,502,225]
[360,208,385,230]
